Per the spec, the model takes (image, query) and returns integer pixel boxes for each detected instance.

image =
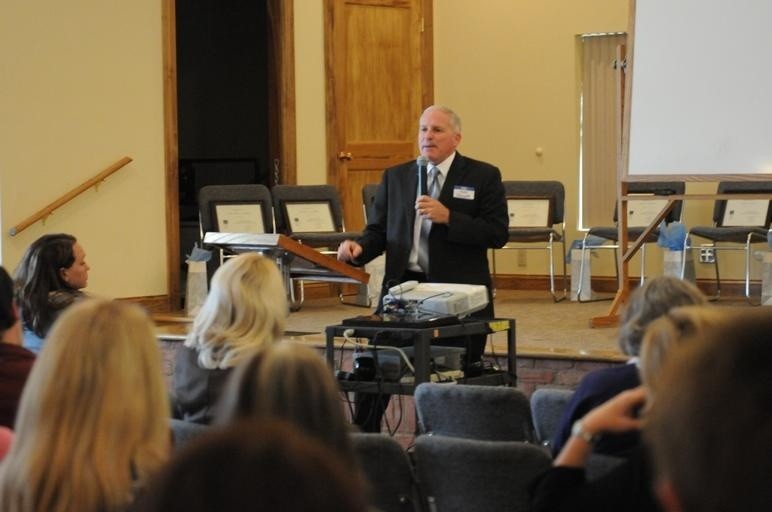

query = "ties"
[417,167,441,277]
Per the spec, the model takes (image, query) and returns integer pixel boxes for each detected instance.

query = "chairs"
[575,179,686,308]
[343,426,416,511]
[406,429,555,512]
[197,183,290,318]
[679,178,771,307]
[487,179,567,304]
[530,383,587,444]
[360,181,382,223]
[414,381,534,446]
[272,184,376,313]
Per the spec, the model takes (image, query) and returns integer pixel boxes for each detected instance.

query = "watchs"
[571,420,604,442]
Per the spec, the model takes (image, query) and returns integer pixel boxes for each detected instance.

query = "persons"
[128,424,381,511]
[338,106,509,451]
[0,260,40,434]
[527,308,771,512]
[551,275,711,467]
[11,233,90,357]
[0,300,172,511]
[217,341,353,472]
[170,253,287,421]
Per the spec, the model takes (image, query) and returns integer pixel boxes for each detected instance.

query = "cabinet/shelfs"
[322,314,517,394]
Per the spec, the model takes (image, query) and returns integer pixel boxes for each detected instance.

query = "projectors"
[382,280,489,319]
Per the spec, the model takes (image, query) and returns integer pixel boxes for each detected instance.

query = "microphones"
[416,155,429,196]
